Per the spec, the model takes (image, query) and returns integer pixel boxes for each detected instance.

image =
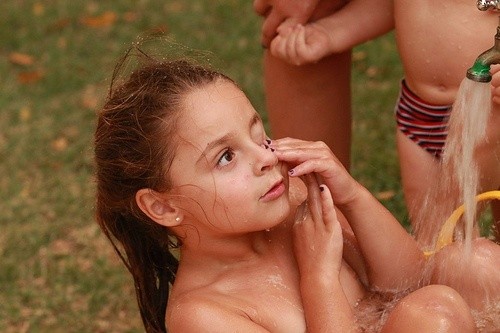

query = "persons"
[251,0,500,247]
[91,28,500,333]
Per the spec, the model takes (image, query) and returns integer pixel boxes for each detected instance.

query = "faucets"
[466,26,500,82]
